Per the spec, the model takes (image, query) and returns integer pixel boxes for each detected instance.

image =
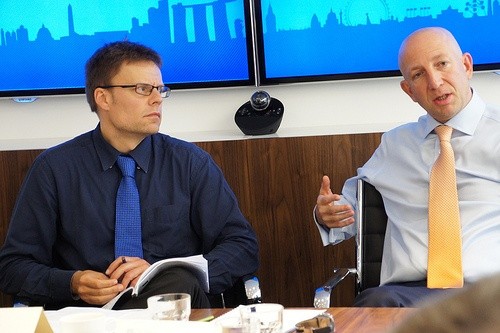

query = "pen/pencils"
[121,256,127,263]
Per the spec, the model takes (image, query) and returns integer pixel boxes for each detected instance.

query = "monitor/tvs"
[0,0,500,98]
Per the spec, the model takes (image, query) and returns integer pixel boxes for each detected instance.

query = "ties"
[426,124,465,288]
[115,155,143,259]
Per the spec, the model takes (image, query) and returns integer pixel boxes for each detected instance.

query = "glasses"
[96,83,171,98]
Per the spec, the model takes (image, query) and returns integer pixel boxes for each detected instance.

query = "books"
[101,253,210,309]
[210,303,327,333]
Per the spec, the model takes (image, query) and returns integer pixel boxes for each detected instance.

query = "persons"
[1,39,262,309]
[311,26,500,308]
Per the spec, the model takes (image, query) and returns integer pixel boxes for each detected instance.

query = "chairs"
[313,179,388,309]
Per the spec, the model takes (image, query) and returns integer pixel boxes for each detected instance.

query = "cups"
[147,293,191,321]
[237,304,284,333]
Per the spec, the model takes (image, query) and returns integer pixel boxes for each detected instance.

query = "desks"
[0,305,418,333]
